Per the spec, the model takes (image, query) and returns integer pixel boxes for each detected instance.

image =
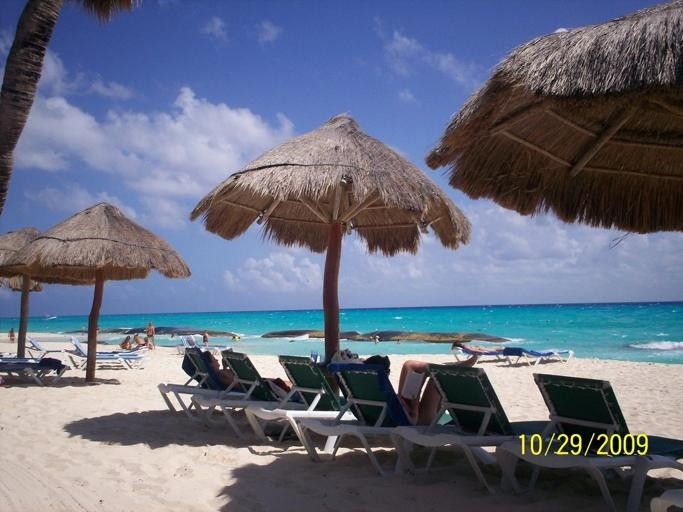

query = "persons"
[131,333,146,352]
[237,337,240,340]
[8,328,16,343]
[452,340,505,353]
[203,330,208,347]
[120,336,132,350]
[203,351,291,393]
[232,336,236,340]
[146,321,155,349]
[364,354,478,427]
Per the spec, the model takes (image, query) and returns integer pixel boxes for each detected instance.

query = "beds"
[450,346,527,367]
[504,347,574,366]
[0,356,60,385]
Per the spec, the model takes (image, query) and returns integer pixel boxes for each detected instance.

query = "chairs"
[244,353,357,462]
[394,362,554,496]
[192,350,287,442]
[72,336,142,370]
[25,335,63,358]
[158,348,248,426]
[504,372,683,512]
[63,348,135,368]
[298,362,453,479]
